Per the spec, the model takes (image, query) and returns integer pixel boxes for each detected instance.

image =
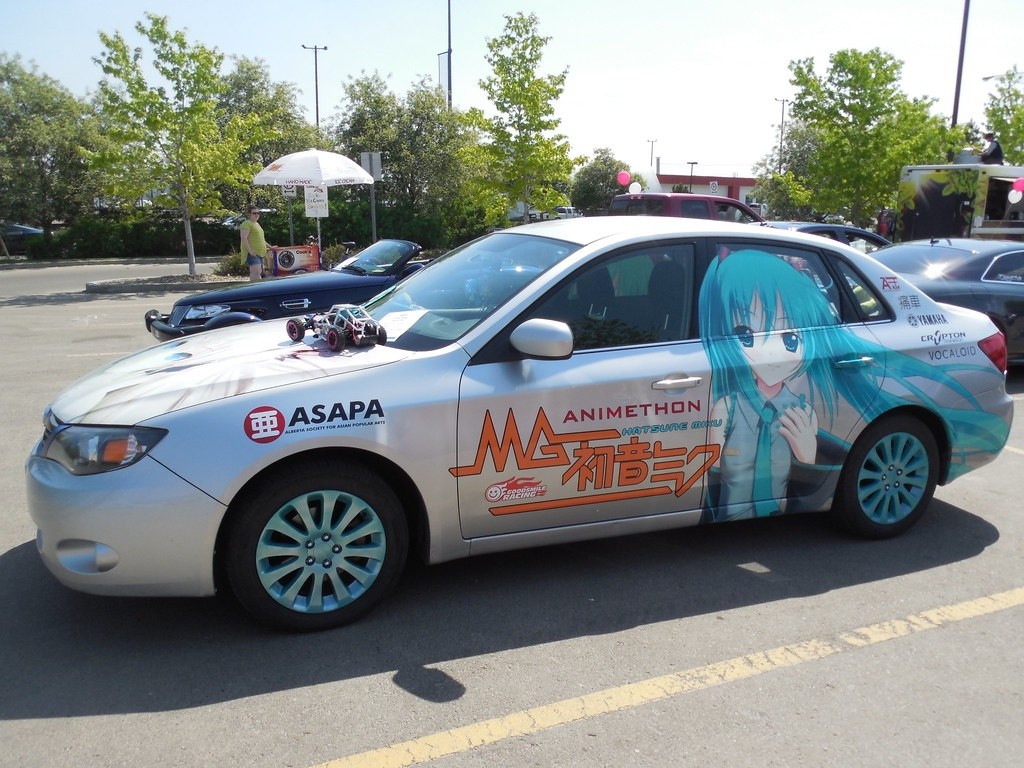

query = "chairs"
[647,259,686,343]
[573,266,616,350]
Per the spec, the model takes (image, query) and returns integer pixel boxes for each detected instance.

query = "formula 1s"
[282,302,388,352]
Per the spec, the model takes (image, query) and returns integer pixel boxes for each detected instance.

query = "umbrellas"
[253,148,375,269]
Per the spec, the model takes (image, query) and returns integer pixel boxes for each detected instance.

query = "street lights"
[688,160,697,194]
[302,43,328,151]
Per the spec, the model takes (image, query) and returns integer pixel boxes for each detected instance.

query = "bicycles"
[288,234,355,277]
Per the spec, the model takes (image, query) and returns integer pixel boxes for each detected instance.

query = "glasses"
[250,212,260,215]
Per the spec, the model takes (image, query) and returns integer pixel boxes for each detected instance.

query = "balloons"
[1008,177,1024,204]
[617,171,641,193]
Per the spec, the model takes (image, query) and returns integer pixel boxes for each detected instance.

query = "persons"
[240,205,278,281]
[974,132,1004,165]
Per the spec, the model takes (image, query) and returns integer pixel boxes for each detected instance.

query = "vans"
[608,193,765,254]
[555,207,584,220]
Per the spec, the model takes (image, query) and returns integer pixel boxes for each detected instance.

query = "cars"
[0,219,53,255]
[142,235,567,345]
[855,236,1024,370]
[742,217,894,280]
[21,214,1014,638]
